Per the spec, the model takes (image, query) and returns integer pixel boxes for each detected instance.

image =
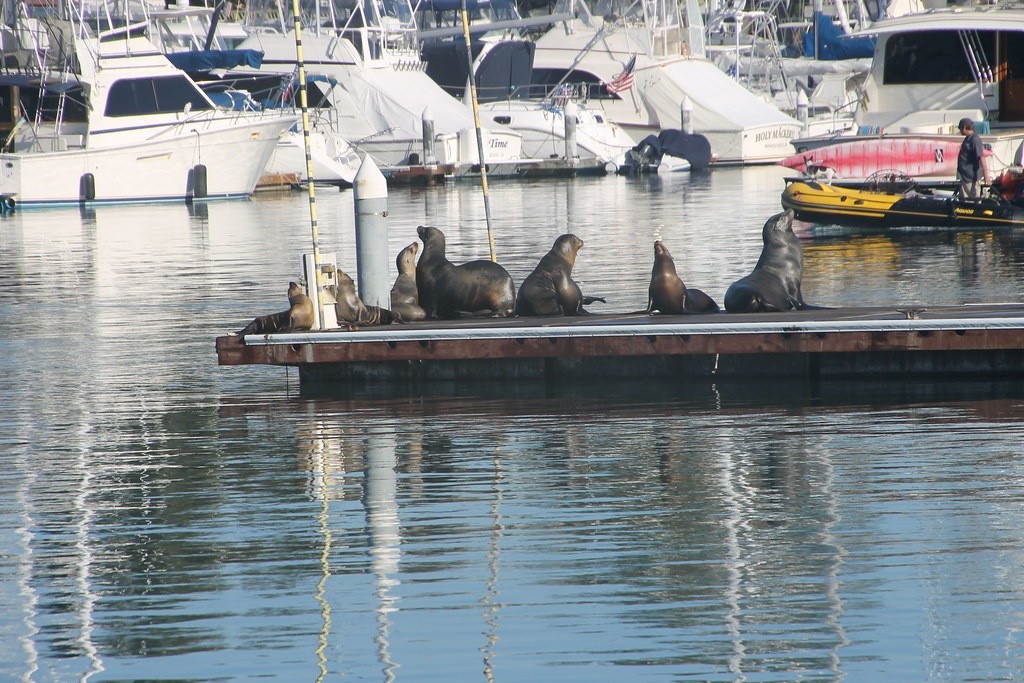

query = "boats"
[1,0,1023,237]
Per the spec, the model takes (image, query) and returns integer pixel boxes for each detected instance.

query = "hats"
[956,118,974,129]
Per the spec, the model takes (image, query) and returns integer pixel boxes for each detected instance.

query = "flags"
[282,64,299,103]
[552,83,573,108]
[606,53,636,92]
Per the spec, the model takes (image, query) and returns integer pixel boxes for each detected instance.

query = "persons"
[955,118,992,204]
